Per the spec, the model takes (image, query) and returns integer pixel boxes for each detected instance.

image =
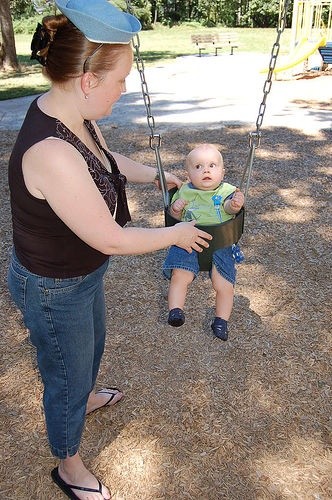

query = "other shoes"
[168,308,185,327]
[211,318,229,341]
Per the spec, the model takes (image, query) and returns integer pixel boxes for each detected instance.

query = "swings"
[124,0,286,272]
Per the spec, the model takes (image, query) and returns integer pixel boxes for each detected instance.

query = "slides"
[259,36,326,81]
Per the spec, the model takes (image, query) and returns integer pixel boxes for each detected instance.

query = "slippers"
[85,388,123,415]
[51,467,110,500]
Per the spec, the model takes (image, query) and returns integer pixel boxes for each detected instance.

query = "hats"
[56,0,142,44]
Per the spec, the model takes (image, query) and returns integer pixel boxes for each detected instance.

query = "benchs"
[190,34,239,57]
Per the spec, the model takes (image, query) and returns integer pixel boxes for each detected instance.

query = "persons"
[159,145,246,340]
[7,0,214,500]
